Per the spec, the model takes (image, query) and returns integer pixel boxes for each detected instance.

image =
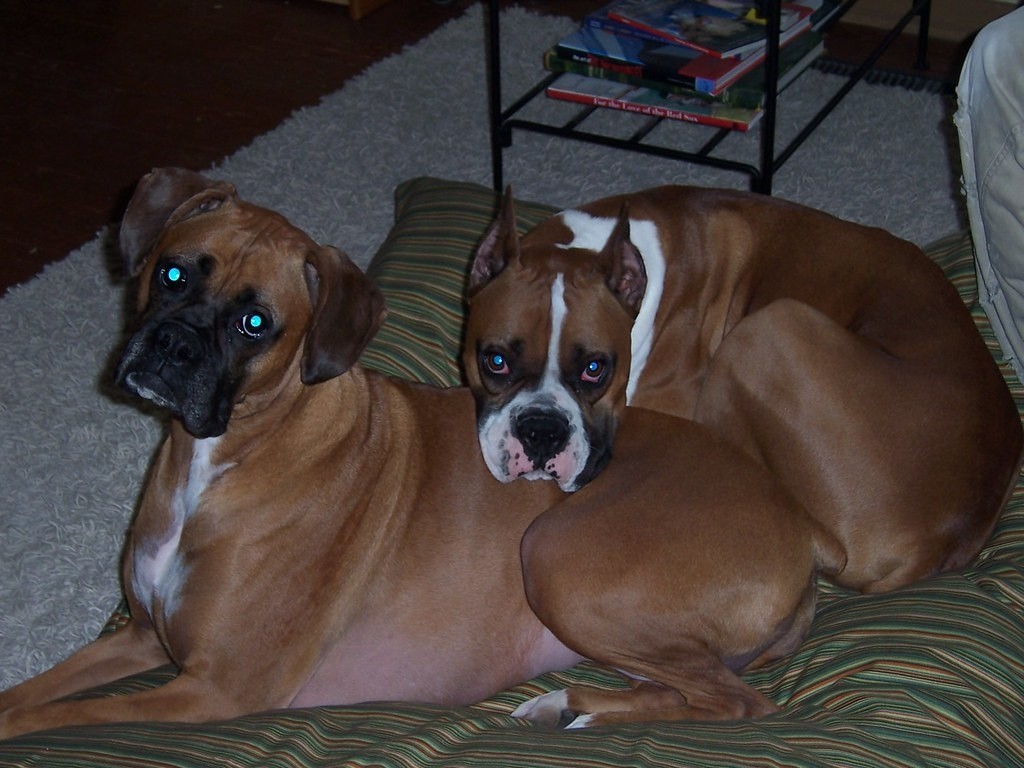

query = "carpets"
[0,0,964,690]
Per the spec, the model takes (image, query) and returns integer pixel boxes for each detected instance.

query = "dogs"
[0,163,1024,738]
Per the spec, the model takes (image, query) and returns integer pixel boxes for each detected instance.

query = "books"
[554,0,813,98]
[545,73,767,133]
[607,0,800,59]
[583,0,825,59]
[544,32,826,109]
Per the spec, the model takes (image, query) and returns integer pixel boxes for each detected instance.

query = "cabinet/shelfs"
[483,0,933,192]
